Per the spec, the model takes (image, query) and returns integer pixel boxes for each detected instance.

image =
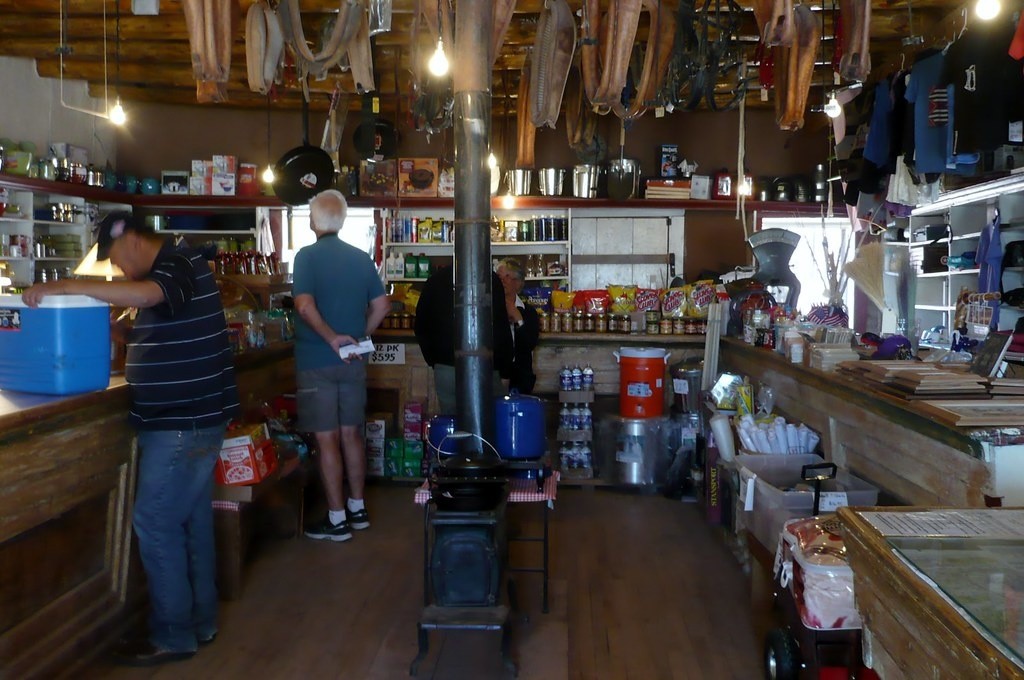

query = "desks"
[211,453,311,601]
[416,469,559,612]
[836,506,1024,680]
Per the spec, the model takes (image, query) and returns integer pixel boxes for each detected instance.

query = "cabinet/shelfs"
[878,175,1024,363]
[0,173,135,297]
[383,208,574,292]
[557,393,595,481]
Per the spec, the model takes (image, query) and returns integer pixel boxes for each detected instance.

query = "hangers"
[899,7,969,73]
[929,212,954,247]
[994,206,1000,222]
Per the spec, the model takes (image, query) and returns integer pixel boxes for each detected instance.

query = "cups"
[28,161,59,179]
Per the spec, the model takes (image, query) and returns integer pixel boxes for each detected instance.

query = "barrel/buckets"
[536,163,601,199]
[503,169,533,195]
[669,361,704,412]
[4,151,33,174]
[422,414,459,464]
[614,346,671,419]
[615,414,656,484]
[494,388,548,459]
[664,407,704,464]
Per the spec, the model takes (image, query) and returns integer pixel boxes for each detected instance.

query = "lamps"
[73,242,130,292]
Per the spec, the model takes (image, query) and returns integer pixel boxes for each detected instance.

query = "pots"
[432,431,511,510]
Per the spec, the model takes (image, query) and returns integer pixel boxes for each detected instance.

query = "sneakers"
[304,511,353,542]
[344,502,371,530]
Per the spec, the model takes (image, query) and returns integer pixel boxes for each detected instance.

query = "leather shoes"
[197,634,216,644]
[111,639,196,666]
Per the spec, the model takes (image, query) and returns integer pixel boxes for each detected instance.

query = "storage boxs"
[735,453,879,554]
[213,401,421,500]
[0,292,110,396]
[158,154,440,195]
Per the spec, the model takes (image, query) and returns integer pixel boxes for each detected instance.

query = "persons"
[21,210,232,664]
[495,258,540,394]
[292,190,391,542]
[415,262,514,415]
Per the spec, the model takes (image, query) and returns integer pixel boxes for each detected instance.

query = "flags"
[807,304,849,328]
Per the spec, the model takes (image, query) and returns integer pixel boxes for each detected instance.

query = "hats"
[97,212,133,261]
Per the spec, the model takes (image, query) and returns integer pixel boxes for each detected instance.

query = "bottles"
[561,403,591,429]
[85,163,106,185]
[561,362,595,389]
[560,441,591,470]
[40,267,58,283]
[36,235,56,257]
[120,174,159,195]
[59,159,85,182]
[215,235,255,252]
[385,253,428,278]
[743,308,763,343]
[525,255,547,276]
[529,214,566,242]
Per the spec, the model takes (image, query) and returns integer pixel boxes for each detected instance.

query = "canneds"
[646,310,708,335]
[517,217,568,242]
[379,312,416,328]
[538,312,631,334]
